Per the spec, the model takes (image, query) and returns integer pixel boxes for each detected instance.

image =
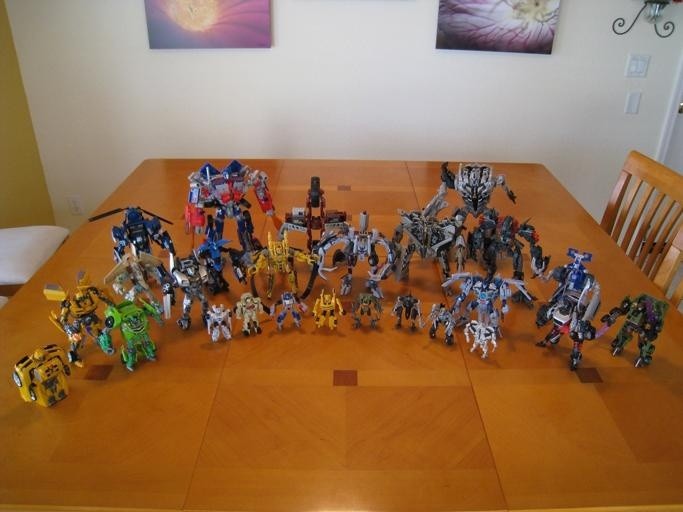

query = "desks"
[1,160,681,510]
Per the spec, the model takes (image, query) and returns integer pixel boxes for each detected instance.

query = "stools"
[0,224,69,295]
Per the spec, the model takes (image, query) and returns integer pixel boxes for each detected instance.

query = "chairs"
[594,148,681,309]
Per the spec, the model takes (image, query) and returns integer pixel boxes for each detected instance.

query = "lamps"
[612,1,677,41]
